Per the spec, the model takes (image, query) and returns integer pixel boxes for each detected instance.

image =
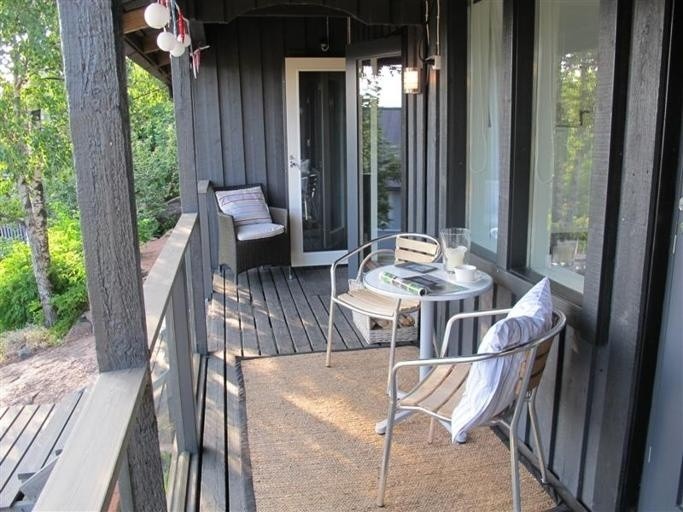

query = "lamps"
[401,66,423,96]
[143,0,192,58]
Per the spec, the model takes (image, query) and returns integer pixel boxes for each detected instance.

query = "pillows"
[213,186,273,228]
[449,274,553,448]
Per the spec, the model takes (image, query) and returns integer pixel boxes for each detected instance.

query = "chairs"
[324,230,441,395]
[375,305,567,510]
[210,182,292,291]
[300,167,320,222]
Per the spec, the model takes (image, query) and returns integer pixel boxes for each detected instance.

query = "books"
[379,271,468,297]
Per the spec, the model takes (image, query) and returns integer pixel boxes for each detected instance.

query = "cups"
[439,227,481,281]
[550,235,585,275]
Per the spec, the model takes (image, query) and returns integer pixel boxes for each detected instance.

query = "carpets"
[233,338,566,512]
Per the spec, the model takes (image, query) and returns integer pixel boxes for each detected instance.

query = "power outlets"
[430,55,440,70]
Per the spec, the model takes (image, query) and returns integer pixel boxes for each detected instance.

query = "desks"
[361,261,495,446]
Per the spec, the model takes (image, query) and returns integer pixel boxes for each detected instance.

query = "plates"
[449,274,484,285]
